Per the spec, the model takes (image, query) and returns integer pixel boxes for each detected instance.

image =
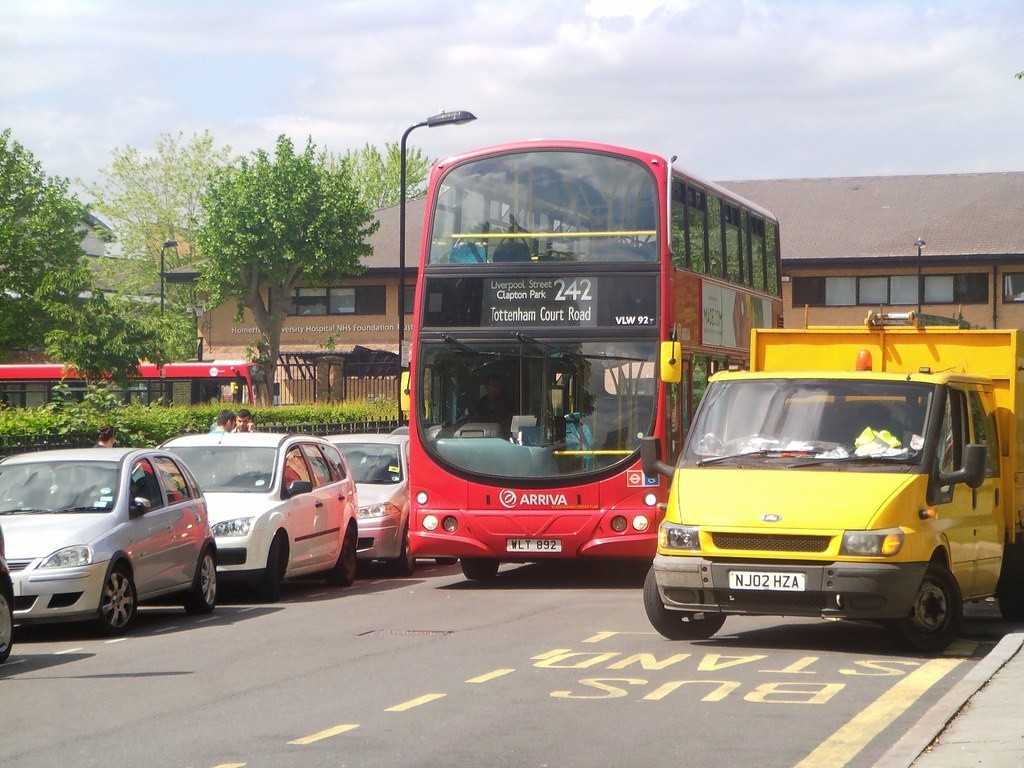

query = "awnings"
[0,360,256,406]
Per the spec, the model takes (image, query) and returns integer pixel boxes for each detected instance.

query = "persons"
[209,410,236,434]
[93,426,116,448]
[459,376,512,424]
[107,475,151,508]
[231,409,257,433]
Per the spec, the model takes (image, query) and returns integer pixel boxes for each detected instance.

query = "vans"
[153,431,358,601]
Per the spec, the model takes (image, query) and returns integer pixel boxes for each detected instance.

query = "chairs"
[851,407,905,451]
[448,221,773,293]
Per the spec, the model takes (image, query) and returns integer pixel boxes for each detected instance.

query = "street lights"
[915,237,925,314]
[159,240,177,404]
[398,109,477,427]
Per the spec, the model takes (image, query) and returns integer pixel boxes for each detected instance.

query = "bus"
[406,140,783,581]
[0,363,274,411]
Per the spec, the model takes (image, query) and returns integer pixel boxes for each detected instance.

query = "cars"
[0,448,217,635]
[323,434,458,580]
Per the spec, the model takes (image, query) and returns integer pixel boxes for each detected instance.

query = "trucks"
[643,303,1024,650]
